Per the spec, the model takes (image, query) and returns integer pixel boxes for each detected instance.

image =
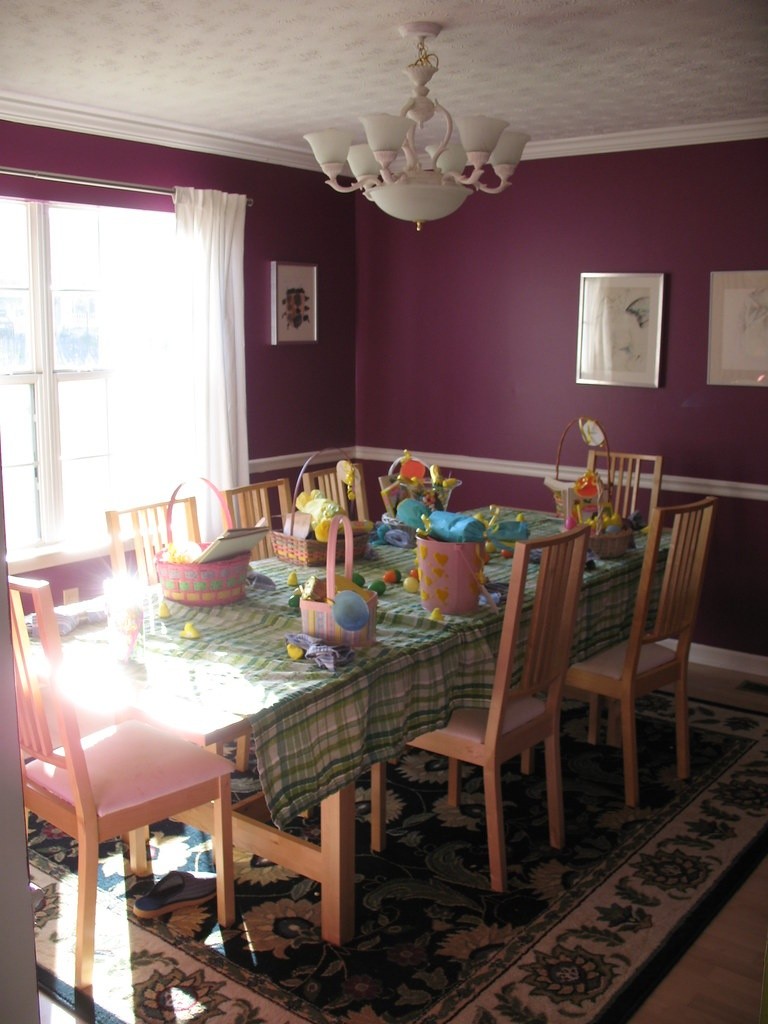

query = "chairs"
[520,496,717,808]
[365,523,593,894]
[105,495,201,582]
[218,479,294,565]
[302,459,367,529]
[6,577,239,988]
[586,450,665,523]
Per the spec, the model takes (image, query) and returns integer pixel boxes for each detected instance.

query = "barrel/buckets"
[415,534,485,615]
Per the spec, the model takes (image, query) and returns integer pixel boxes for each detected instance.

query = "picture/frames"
[574,270,664,392]
[271,261,319,346]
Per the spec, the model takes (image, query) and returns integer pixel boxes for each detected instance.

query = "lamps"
[301,19,531,230]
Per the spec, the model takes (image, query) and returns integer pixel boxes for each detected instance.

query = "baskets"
[267,446,370,568]
[296,514,378,648]
[388,455,463,511]
[155,476,252,607]
[382,511,417,539]
[544,416,612,519]
[588,526,632,559]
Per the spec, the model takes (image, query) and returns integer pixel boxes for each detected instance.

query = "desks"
[27,504,714,945]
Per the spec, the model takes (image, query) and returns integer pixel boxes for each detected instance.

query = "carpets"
[23,689,767,1024]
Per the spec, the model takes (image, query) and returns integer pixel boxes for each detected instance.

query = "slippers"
[132,869,217,919]
